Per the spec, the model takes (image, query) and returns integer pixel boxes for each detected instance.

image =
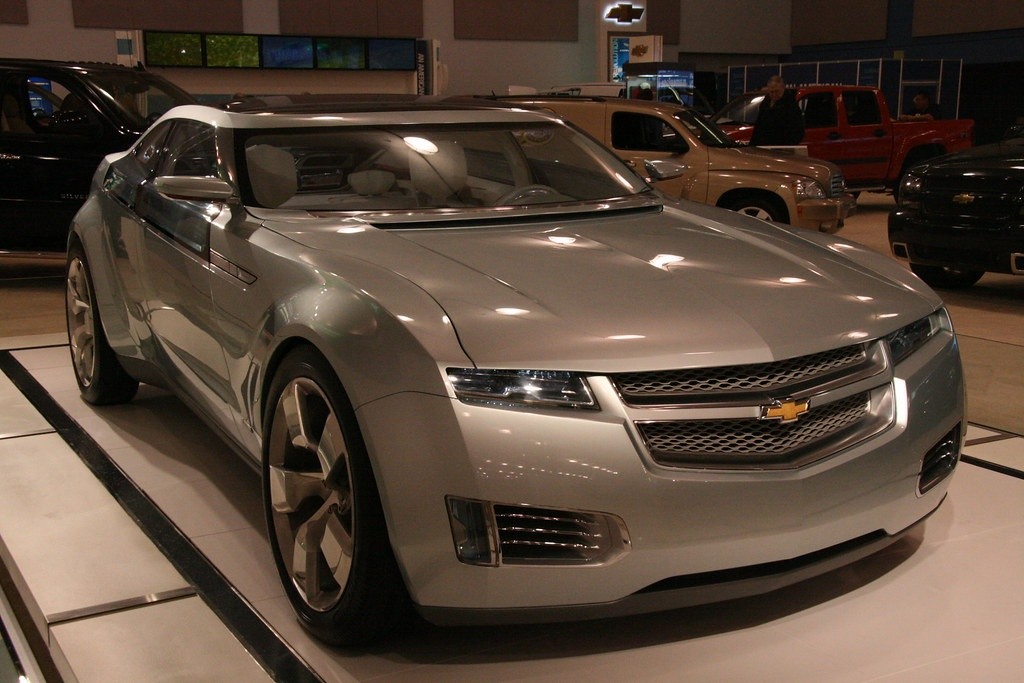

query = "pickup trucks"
[702,84,976,205]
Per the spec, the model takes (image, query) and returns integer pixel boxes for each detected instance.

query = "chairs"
[408,139,478,208]
[246,145,298,209]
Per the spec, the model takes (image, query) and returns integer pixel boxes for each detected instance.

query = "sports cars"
[61,96,968,645]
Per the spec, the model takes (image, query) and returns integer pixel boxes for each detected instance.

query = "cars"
[435,83,858,235]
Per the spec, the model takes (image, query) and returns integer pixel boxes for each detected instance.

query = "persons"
[748,76,805,149]
[898,89,943,121]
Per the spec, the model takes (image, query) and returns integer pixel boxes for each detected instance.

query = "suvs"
[888,124,1024,289]
[0,57,365,253]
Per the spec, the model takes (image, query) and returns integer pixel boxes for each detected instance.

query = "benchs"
[286,166,426,215]
